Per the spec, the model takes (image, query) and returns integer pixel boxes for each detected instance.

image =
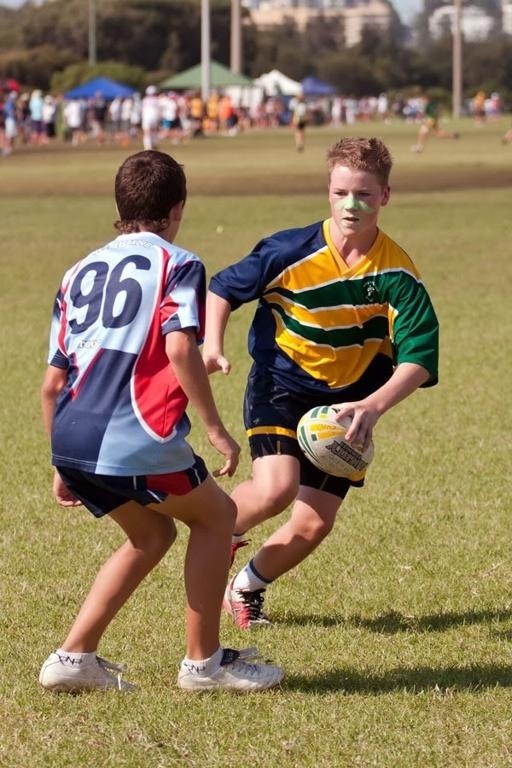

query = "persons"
[36,145,286,695]
[194,137,441,630]
[0,59,511,165]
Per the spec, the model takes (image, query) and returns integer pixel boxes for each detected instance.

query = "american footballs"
[298,406,375,480]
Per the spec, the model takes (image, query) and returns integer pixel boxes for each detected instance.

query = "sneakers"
[220,574,273,636]
[177,647,284,691]
[38,652,139,695]
